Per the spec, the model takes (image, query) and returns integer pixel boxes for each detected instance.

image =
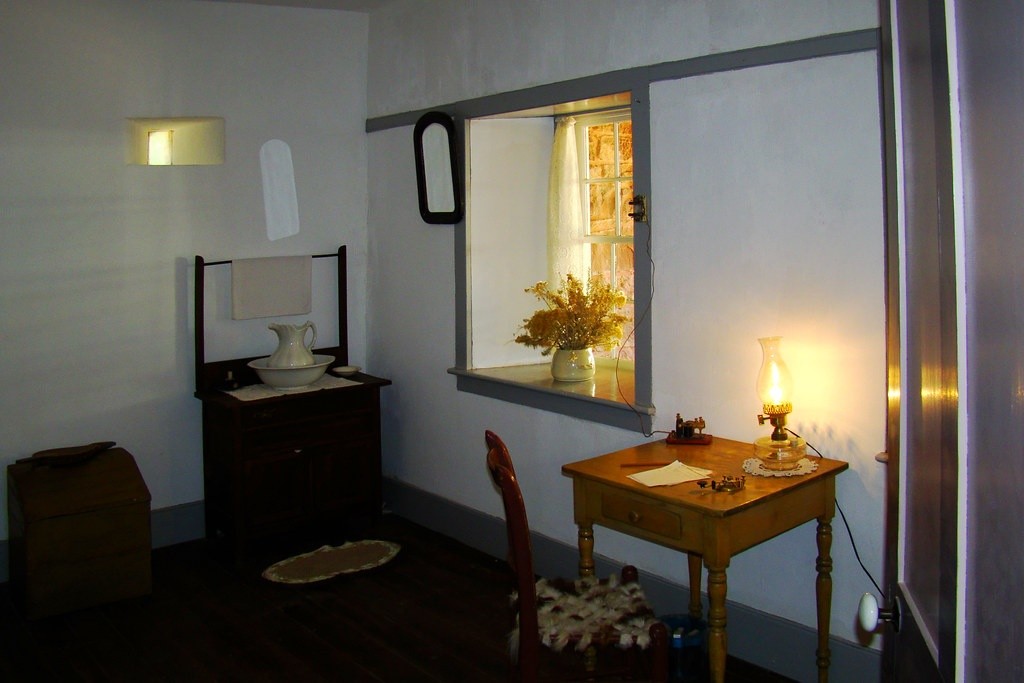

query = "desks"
[561,434,849,683]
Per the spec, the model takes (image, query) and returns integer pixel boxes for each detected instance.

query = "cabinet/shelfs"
[193,245,393,560]
[6,438,154,621]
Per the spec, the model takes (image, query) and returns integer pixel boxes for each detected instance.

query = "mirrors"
[413,109,461,223]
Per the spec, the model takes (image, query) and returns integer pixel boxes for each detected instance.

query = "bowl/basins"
[248,355,336,390]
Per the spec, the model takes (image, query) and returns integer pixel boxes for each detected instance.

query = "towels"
[230,253,313,321]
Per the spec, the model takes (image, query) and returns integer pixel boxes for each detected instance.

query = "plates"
[334,366,361,375]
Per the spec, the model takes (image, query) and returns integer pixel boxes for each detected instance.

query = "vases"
[551,346,596,382]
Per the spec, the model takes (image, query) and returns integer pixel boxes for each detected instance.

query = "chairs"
[485,430,669,683]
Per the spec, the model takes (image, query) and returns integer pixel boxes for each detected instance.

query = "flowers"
[513,273,632,350]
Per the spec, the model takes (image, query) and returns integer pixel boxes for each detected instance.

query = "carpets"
[256,536,401,589]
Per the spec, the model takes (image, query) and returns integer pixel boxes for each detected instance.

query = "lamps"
[753,336,806,472]
[123,116,225,165]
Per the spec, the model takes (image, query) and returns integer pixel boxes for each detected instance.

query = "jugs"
[267,320,318,368]
